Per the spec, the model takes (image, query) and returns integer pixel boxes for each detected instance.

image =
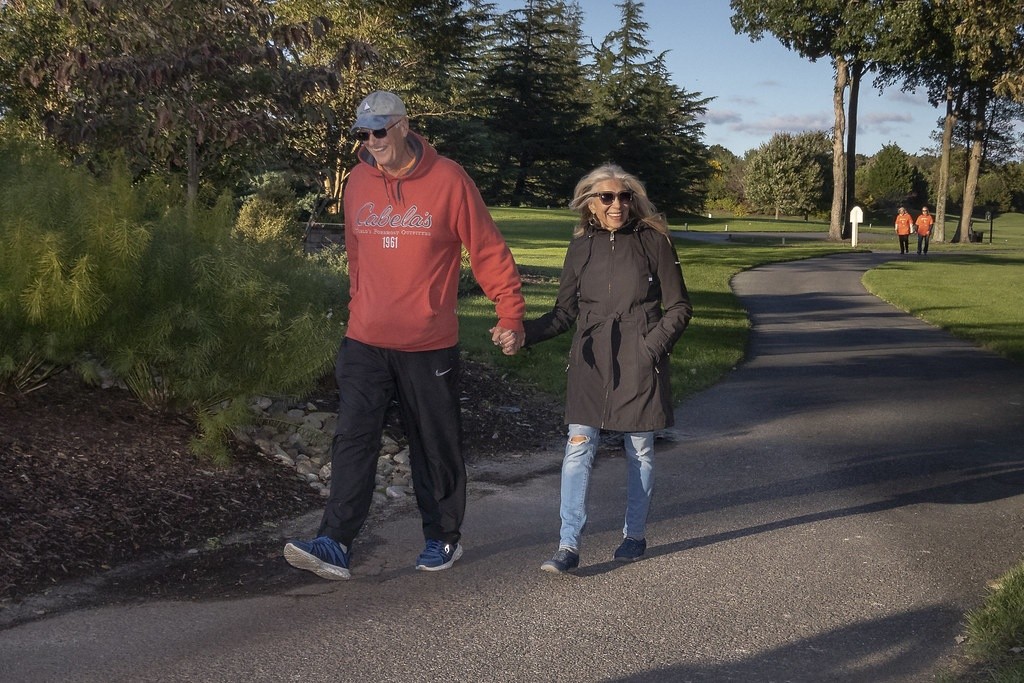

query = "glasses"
[351,117,404,140]
[585,190,634,206]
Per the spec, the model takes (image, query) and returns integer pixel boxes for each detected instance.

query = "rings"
[500,340,504,345]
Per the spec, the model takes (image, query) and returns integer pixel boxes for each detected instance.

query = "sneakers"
[538,546,580,576]
[614,535,647,562]
[412,537,466,573]
[282,537,353,583]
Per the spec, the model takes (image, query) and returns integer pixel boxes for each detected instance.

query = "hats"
[350,90,407,133]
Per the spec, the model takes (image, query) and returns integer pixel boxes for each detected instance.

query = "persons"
[282,89,526,581]
[488,164,694,575]
[914,207,934,256]
[894,206,914,254]
